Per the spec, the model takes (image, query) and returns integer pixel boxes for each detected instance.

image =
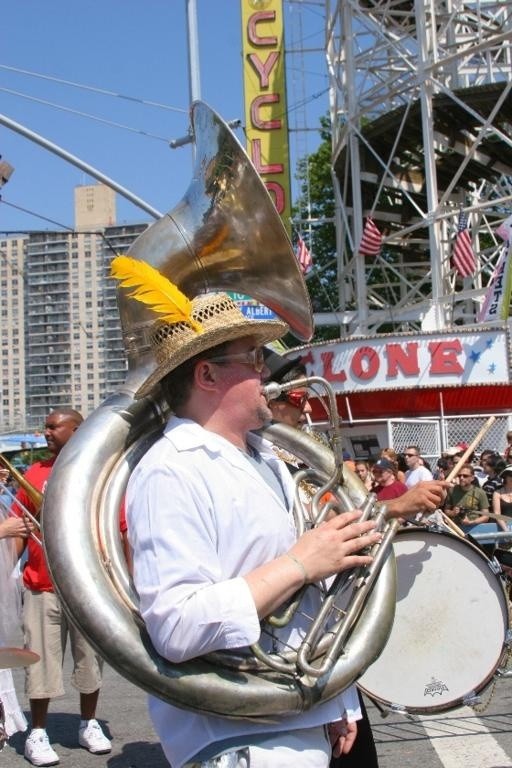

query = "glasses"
[286,393,309,408]
[402,453,417,457]
[206,347,265,374]
[457,474,473,478]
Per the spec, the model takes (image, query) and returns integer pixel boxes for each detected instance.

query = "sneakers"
[24,730,60,767]
[78,718,113,754]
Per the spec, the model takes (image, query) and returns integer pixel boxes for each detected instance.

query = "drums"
[320,526,511,714]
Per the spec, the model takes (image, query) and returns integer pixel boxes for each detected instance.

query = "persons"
[1,410,145,768]
[314,430,512,646]
[129,294,459,767]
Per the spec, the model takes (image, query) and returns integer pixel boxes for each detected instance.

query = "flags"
[358,215,382,257]
[296,237,313,276]
[453,208,477,280]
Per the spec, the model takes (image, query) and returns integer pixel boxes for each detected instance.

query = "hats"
[373,458,396,471]
[497,464,512,479]
[263,346,303,383]
[134,292,290,400]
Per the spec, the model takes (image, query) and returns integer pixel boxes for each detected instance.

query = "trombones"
[1,456,43,545]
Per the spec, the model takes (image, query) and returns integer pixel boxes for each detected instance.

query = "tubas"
[38,101,405,721]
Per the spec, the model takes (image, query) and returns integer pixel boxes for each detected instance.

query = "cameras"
[459,506,469,514]
